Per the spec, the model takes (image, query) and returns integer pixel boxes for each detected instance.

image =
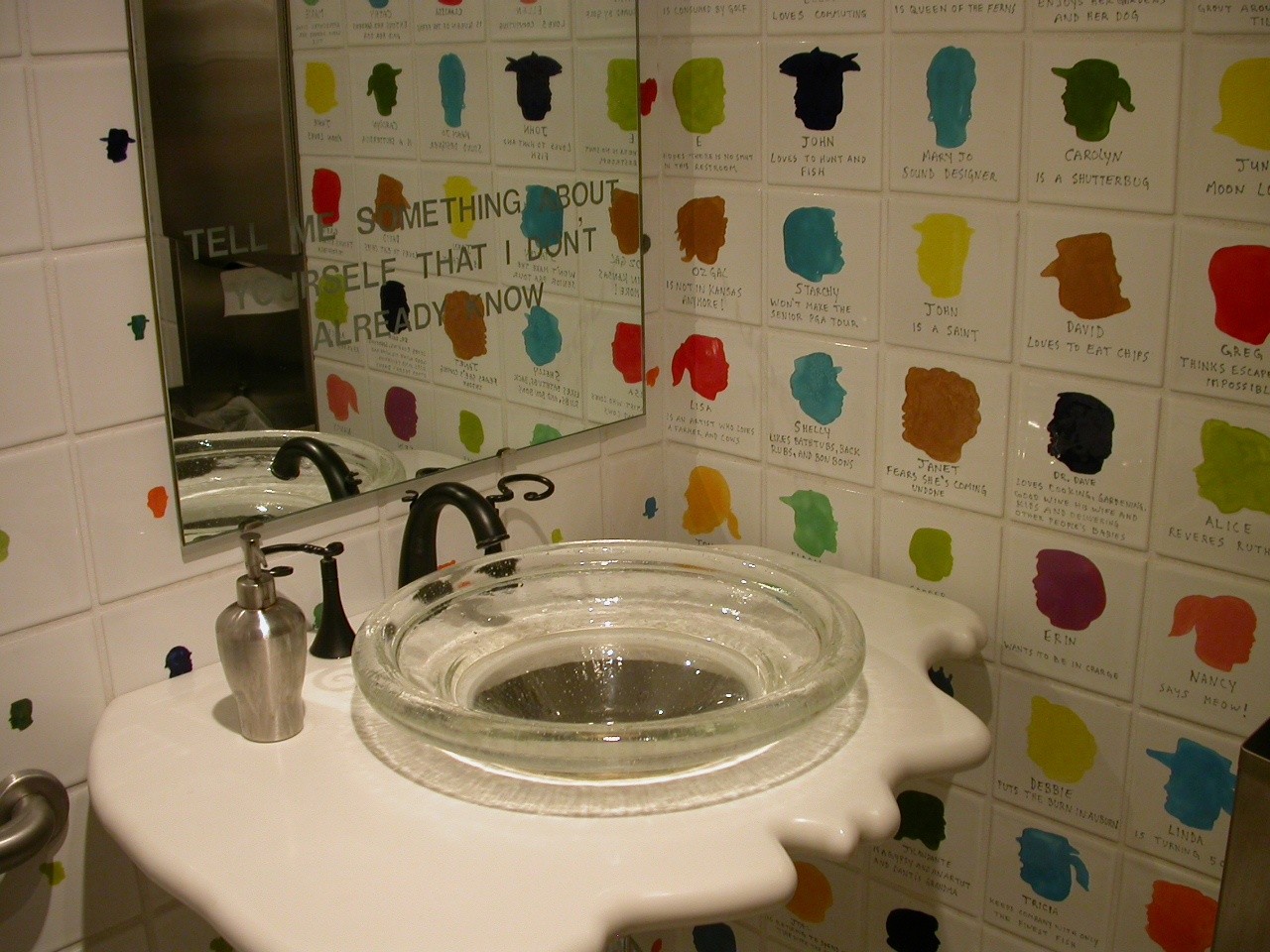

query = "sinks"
[349,538,866,783]
[172,429,407,546]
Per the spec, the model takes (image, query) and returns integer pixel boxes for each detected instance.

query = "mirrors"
[126,0,646,545]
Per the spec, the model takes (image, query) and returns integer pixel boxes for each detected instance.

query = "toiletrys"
[215,532,308,744]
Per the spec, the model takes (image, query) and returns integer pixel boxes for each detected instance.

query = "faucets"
[270,436,361,502]
[398,481,510,589]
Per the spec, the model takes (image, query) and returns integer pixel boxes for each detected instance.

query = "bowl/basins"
[350,536,866,777]
[172,431,402,544]
